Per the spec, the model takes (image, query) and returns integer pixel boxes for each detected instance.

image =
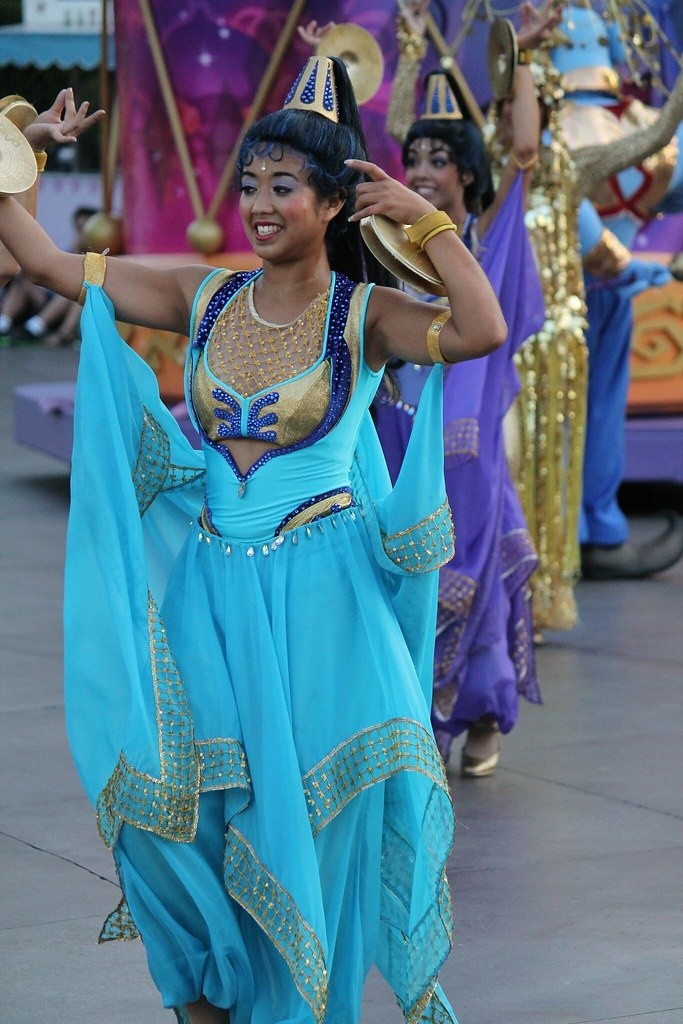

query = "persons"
[297,0,568,776]
[0,88,106,275]
[382,1,683,645]
[0,209,96,348]
[0,55,509,1024]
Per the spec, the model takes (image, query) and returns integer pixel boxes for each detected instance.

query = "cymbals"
[358,212,448,298]
[0,112,39,195]
[487,16,519,101]
[313,22,386,105]
[371,214,444,287]
[0,94,39,133]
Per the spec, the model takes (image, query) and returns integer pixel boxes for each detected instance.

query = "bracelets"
[34,151,47,172]
[519,49,531,64]
[404,211,457,250]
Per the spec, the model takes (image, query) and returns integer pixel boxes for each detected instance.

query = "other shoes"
[460,744,502,778]
[533,632,544,646]
[432,748,451,767]
[37,331,78,349]
[0,324,36,343]
